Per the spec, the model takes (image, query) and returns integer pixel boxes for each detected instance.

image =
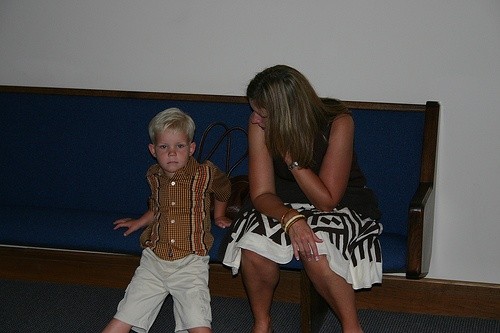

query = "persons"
[102,107,233,333]
[222,65,382,333]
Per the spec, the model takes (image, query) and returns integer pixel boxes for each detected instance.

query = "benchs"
[0,85,441,333]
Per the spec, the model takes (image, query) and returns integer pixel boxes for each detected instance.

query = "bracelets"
[280,208,306,233]
[288,160,299,172]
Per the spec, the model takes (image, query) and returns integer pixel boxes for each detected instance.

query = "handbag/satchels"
[196,122,249,218]
